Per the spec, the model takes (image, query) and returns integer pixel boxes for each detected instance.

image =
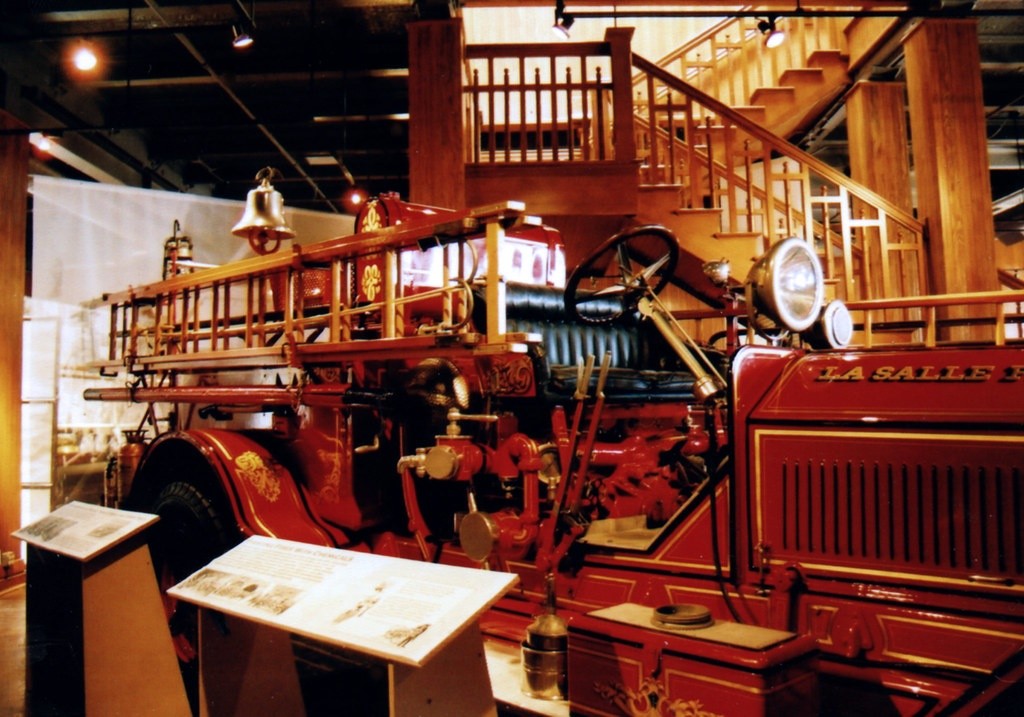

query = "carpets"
[757,18,786,50]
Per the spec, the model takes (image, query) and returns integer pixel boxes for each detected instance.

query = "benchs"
[471,280,703,404]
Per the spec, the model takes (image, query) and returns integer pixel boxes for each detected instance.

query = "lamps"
[553,14,575,41]
[229,24,253,48]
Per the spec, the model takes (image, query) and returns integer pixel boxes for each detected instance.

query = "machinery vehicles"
[80,197,1024,717]
